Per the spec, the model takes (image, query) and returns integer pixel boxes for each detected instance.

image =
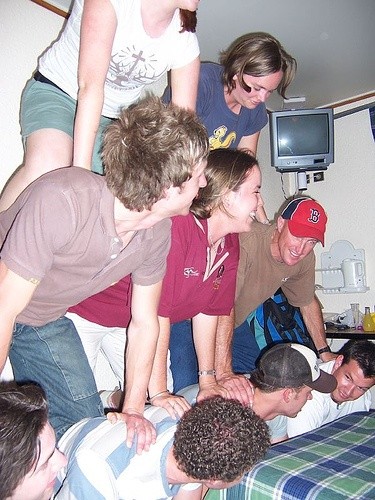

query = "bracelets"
[149,390,169,400]
[199,370,215,375]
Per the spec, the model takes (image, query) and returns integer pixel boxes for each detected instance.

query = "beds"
[201,408,375,499]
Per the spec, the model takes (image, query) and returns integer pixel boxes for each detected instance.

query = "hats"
[258,343,338,393]
[281,197,327,248]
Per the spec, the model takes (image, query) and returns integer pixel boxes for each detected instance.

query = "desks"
[325,326,375,340]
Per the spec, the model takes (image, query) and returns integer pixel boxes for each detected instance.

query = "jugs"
[341,259,364,287]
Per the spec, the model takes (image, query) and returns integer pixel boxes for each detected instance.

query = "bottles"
[362,307,374,330]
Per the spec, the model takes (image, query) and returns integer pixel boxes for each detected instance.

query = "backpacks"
[246,292,321,360]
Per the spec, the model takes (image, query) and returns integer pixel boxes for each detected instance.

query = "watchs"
[319,345,332,354]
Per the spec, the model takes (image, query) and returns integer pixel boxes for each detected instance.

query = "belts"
[33,70,118,122]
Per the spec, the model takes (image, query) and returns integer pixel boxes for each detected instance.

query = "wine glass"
[350,302,360,333]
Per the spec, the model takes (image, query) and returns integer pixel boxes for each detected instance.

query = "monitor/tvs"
[269,109,334,172]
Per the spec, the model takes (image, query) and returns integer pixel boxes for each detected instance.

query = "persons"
[1,92,211,455]
[0,378,67,500]
[162,30,298,225]
[175,341,338,445]
[169,202,343,408]
[1,0,200,214]
[62,147,263,420]
[285,337,375,438]
[50,394,274,500]
